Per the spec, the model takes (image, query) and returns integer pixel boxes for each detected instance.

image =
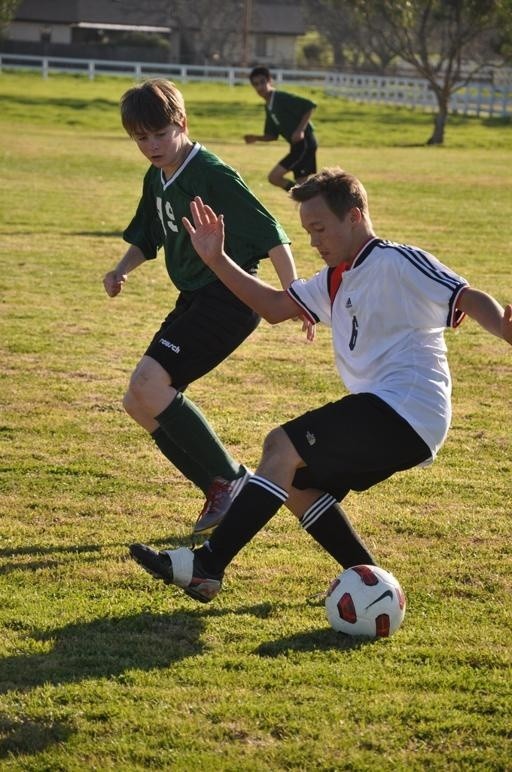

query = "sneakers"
[123,541,224,604]
[193,463,252,533]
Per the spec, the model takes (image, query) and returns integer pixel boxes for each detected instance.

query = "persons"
[102,78,317,538]
[241,66,318,212]
[128,168,512,605]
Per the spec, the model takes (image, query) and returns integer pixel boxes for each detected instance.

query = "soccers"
[327,565,407,638]
